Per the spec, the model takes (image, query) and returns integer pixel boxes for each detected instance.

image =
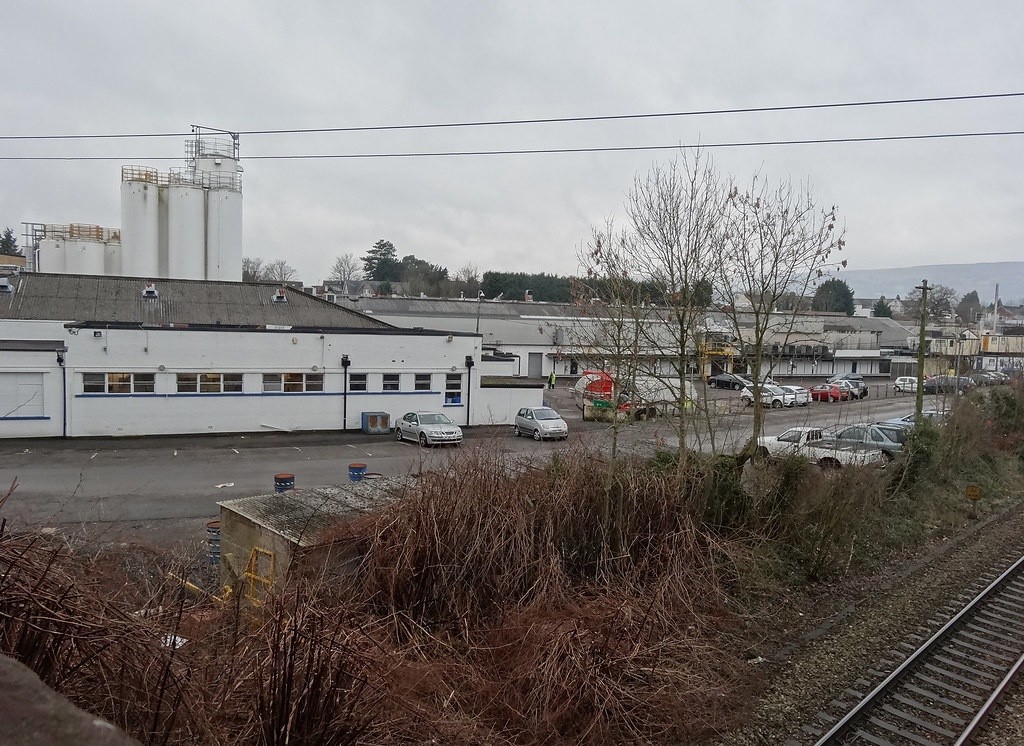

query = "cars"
[924,371,1011,395]
[820,422,909,464]
[874,408,955,438]
[737,372,781,387]
[892,376,924,393]
[806,383,850,403]
[778,385,813,407]
[739,382,796,409]
[394,410,464,448]
[707,372,754,391]
[826,372,864,384]
[514,406,569,441]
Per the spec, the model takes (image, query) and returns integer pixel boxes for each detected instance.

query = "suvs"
[831,380,870,400]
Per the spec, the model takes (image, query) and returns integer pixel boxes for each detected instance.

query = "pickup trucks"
[750,426,883,480]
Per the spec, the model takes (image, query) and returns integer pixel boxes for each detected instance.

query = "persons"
[547,372,555,392]
[946,367,955,376]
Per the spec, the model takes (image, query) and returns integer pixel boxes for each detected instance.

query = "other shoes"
[552,389,555,392]
[547,388,550,391]
